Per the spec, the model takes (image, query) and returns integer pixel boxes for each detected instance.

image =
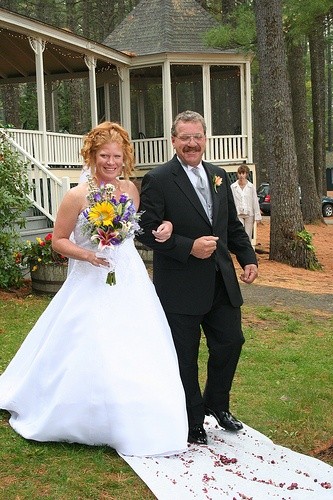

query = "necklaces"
[94,175,120,191]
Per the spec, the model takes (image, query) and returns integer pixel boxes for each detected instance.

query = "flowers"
[211,173,224,193]
[15,234,69,268]
[85,178,146,286]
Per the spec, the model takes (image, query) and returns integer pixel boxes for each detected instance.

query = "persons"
[229,164,260,244]
[50,121,174,458]
[133,110,259,446]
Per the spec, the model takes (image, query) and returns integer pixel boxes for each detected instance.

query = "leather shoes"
[203,398,243,431]
[188,425,208,445]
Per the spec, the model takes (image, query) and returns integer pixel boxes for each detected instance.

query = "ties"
[191,167,215,223]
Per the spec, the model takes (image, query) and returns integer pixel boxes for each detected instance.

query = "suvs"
[257,183,333,217]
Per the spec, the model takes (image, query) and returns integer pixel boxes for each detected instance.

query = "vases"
[30,263,68,293]
